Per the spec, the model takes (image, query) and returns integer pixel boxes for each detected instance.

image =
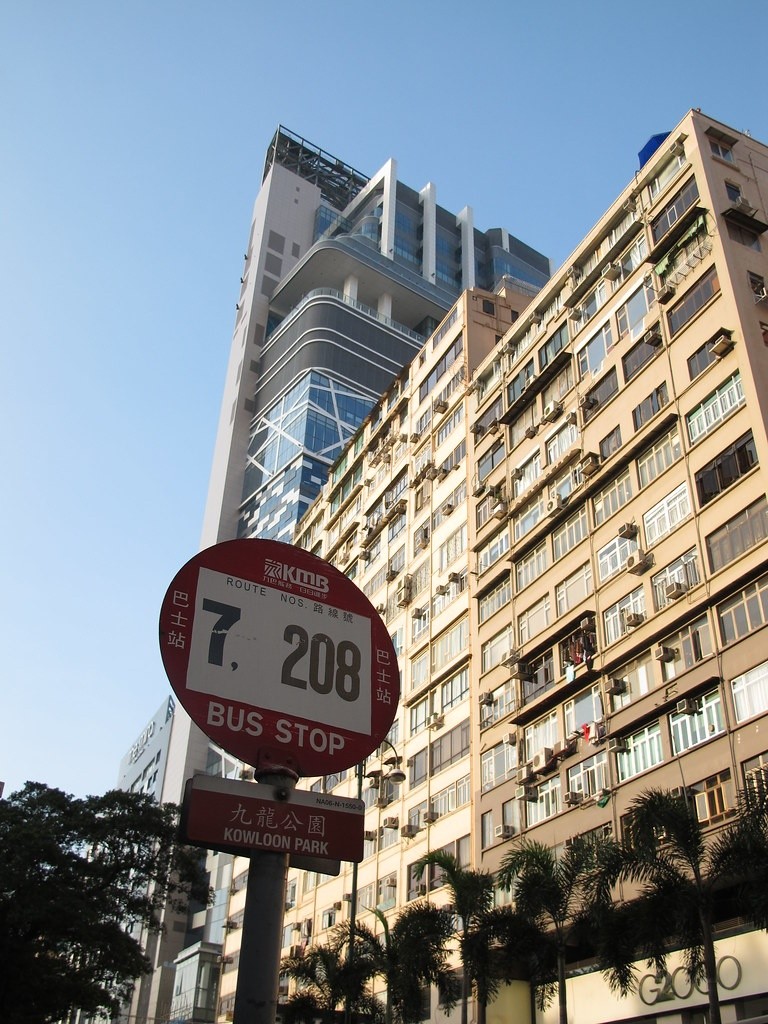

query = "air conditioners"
[210,136,768,1024]
